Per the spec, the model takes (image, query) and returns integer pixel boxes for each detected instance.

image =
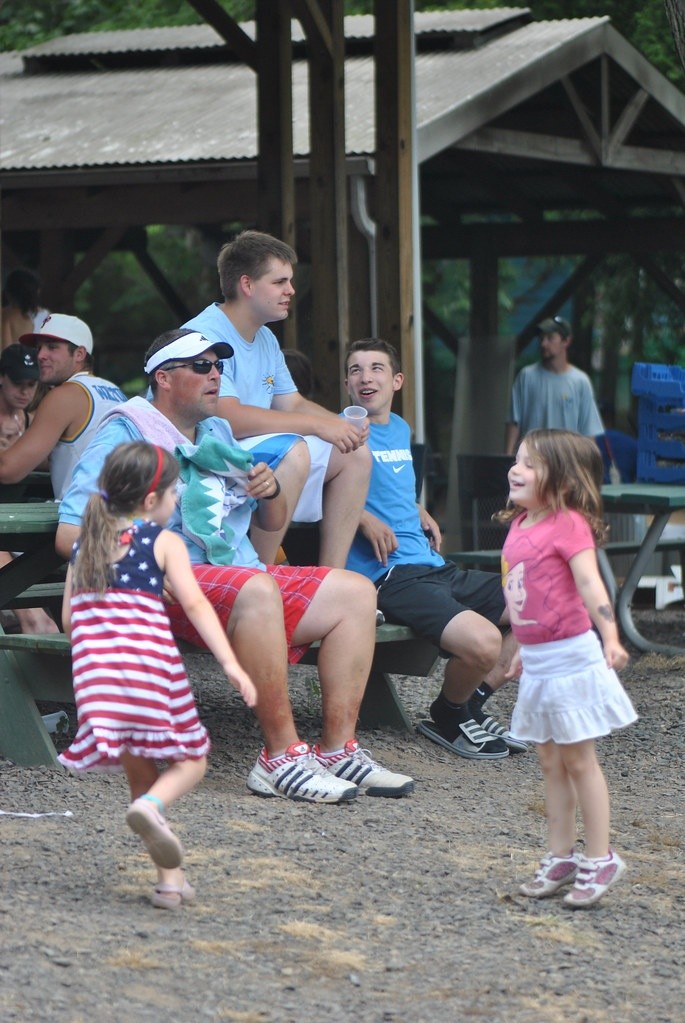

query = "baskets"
[630,360,685,485]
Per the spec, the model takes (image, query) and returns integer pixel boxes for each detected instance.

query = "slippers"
[417,717,528,760]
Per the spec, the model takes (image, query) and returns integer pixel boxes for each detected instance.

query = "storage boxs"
[632,363,685,483]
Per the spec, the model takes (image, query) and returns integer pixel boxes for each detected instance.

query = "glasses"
[554,316,564,329]
[164,360,223,374]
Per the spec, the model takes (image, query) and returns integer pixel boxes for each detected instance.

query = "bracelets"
[263,476,280,499]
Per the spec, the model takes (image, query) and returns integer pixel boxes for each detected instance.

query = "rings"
[265,481,269,485]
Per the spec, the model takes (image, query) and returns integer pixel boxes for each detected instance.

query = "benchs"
[446,537,684,564]
[0,582,417,654]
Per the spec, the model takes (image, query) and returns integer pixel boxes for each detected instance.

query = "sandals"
[126,798,183,869]
[151,878,194,908]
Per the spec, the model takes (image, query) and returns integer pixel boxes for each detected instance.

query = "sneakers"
[245,741,358,804]
[311,739,414,798]
[519,849,627,906]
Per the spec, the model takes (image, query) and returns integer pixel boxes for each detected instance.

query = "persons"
[147,230,372,571]
[336,338,531,759]
[506,317,606,456]
[501,429,640,908]
[0,267,40,352]
[0,312,129,636]
[56,325,416,804]
[56,440,257,909]
[0,343,49,473]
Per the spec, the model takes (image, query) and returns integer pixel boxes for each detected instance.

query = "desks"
[0,473,51,501]
[0,500,412,771]
[590,484,685,656]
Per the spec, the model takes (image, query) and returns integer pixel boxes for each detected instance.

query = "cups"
[343,405,368,431]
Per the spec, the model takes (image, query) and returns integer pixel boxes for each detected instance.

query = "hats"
[143,330,233,374]
[537,317,572,338]
[0,344,38,380]
[17,313,93,356]
